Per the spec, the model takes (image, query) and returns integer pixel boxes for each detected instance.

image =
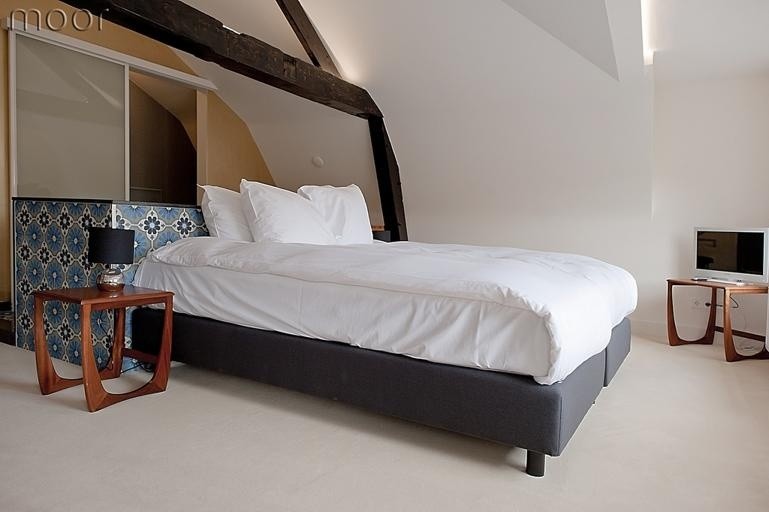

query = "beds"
[128,234,644,481]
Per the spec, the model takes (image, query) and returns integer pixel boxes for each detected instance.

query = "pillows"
[235,173,335,249]
[193,178,254,242]
[294,179,377,245]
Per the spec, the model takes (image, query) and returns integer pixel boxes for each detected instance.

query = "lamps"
[371,229,392,246]
[82,224,140,293]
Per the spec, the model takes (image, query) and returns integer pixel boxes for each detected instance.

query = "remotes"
[691,276,708,281]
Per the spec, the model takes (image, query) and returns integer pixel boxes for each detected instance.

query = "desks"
[664,277,768,364]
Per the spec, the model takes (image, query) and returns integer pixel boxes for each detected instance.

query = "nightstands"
[30,286,178,414]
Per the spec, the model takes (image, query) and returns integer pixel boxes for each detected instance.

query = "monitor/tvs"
[691,227,769,285]
